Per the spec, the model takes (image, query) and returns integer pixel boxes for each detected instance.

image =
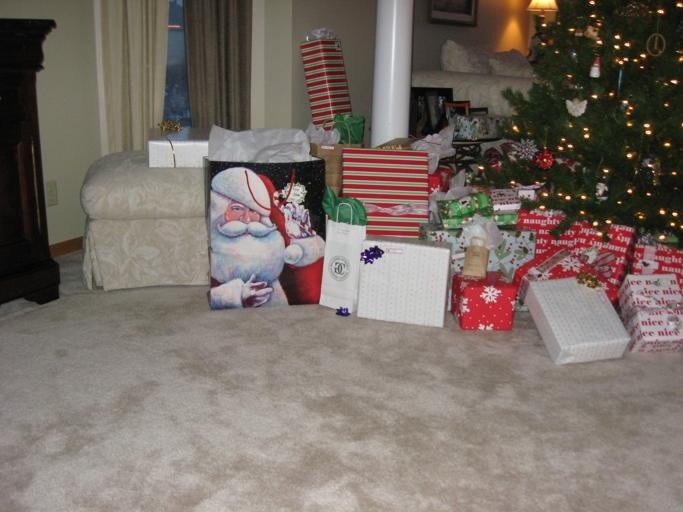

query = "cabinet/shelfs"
[0,19,62,313]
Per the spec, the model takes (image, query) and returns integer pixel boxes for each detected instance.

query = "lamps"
[526,0,560,24]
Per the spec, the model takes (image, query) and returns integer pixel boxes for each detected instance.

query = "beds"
[80,38,546,288]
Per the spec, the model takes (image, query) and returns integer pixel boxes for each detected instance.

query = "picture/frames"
[427,1,479,27]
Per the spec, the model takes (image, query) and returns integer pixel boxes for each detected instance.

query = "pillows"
[431,37,541,79]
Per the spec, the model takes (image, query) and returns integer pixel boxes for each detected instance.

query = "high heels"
[316,201,368,316]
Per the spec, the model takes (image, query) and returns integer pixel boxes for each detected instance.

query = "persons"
[209,166,301,310]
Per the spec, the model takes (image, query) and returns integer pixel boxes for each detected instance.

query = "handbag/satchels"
[202,149,329,309]
[460,235,490,281]
